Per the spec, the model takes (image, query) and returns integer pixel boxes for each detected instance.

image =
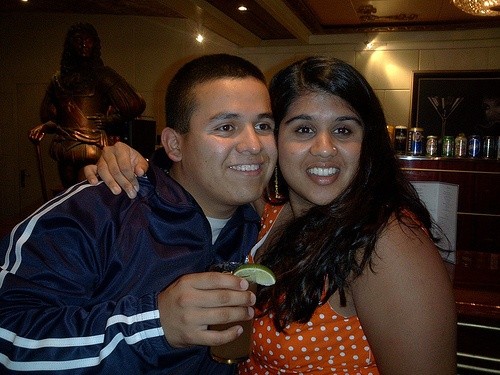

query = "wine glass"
[428,96,463,137]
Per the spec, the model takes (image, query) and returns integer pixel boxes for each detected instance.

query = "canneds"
[425,136,438,157]
[469,135,480,157]
[395,125,408,155]
[482,136,495,158]
[407,128,425,156]
[455,133,467,157]
[386,124,394,147]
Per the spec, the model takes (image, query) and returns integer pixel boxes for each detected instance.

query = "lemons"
[233,263,276,286]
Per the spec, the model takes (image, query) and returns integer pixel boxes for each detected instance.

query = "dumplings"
[441,136,454,157]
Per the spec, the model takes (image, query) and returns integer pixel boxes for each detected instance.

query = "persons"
[83,55,458,375]
[0,53,279,375]
[26,21,147,188]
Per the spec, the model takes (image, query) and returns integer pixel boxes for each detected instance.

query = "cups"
[208,261,257,364]
